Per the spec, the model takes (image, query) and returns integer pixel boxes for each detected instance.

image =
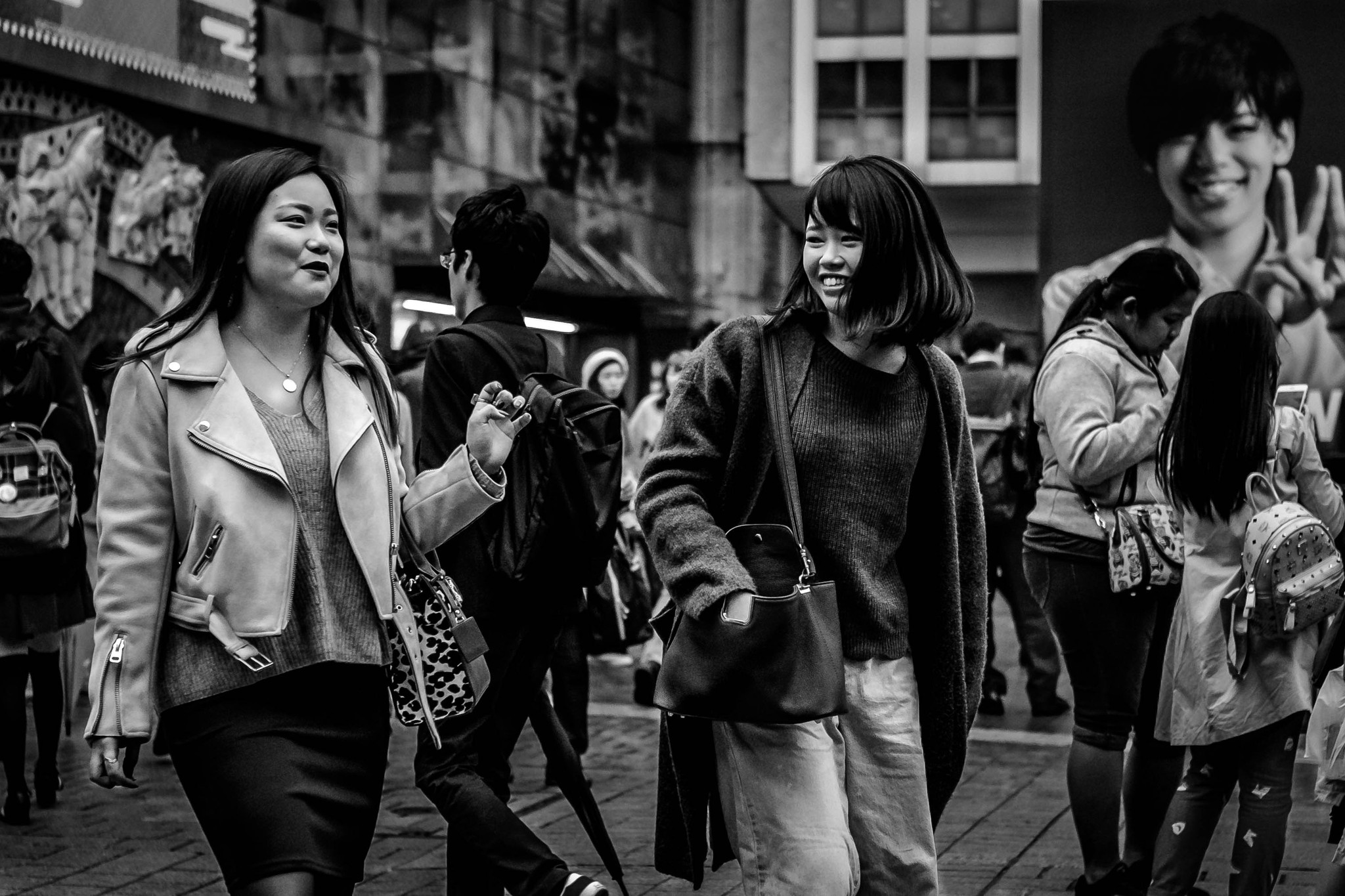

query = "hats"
[581,346,630,392]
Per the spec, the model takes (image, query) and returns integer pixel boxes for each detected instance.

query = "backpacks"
[436,321,624,595]
[976,371,1044,579]
[1219,406,1344,685]
[0,402,83,552]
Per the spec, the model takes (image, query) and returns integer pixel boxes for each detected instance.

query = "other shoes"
[1075,863,1210,896]
[1031,693,1072,717]
[632,660,662,707]
[978,692,1006,716]
[1,792,31,822]
[588,652,636,666]
[561,872,608,896]
[32,769,64,810]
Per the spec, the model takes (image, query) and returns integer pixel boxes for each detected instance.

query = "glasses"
[440,250,459,270]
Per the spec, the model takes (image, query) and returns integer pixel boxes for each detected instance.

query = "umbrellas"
[529,686,630,896]
[63,626,77,737]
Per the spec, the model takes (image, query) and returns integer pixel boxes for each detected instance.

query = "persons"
[83,148,533,896]
[1021,247,1201,896]
[634,154,988,896]
[1042,16,1345,481]
[414,183,725,896]
[1147,291,1345,896]
[0,237,170,827]
[945,322,1075,717]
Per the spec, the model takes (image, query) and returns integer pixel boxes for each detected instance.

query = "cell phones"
[1273,383,1308,414]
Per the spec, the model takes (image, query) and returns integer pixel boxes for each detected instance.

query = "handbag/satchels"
[1107,503,1186,597]
[383,499,491,751]
[647,524,849,724]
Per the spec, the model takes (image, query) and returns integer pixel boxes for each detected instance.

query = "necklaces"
[232,319,311,392]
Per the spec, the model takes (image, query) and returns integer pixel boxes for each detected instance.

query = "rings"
[99,751,116,765]
[470,394,490,405]
